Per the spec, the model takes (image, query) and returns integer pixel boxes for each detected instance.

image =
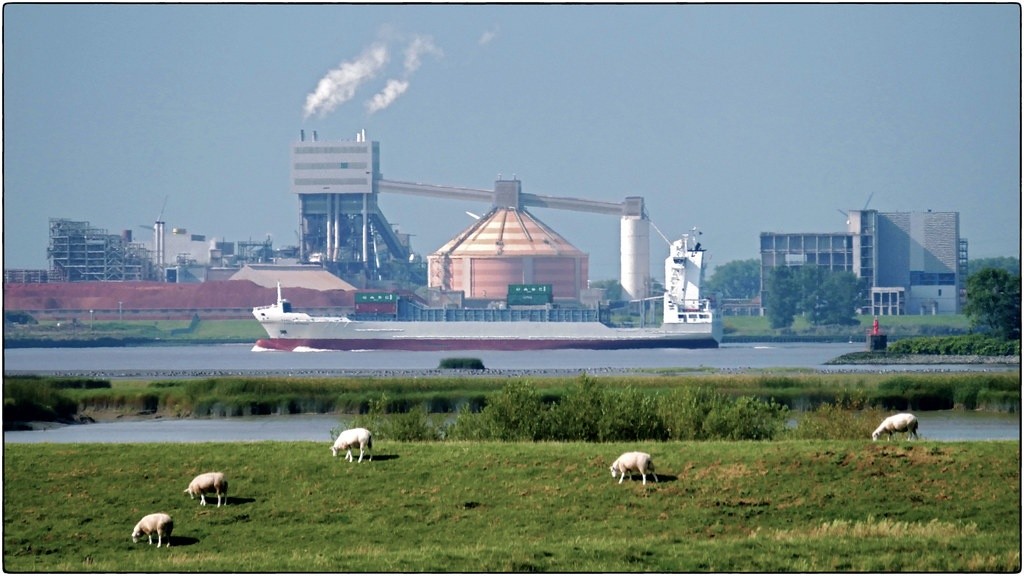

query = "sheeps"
[329,428,373,464]
[872,413,919,441]
[610,451,658,486]
[184,471,228,508]
[131,513,173,548]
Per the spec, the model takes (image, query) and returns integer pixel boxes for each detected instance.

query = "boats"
[251,225,726,351]
